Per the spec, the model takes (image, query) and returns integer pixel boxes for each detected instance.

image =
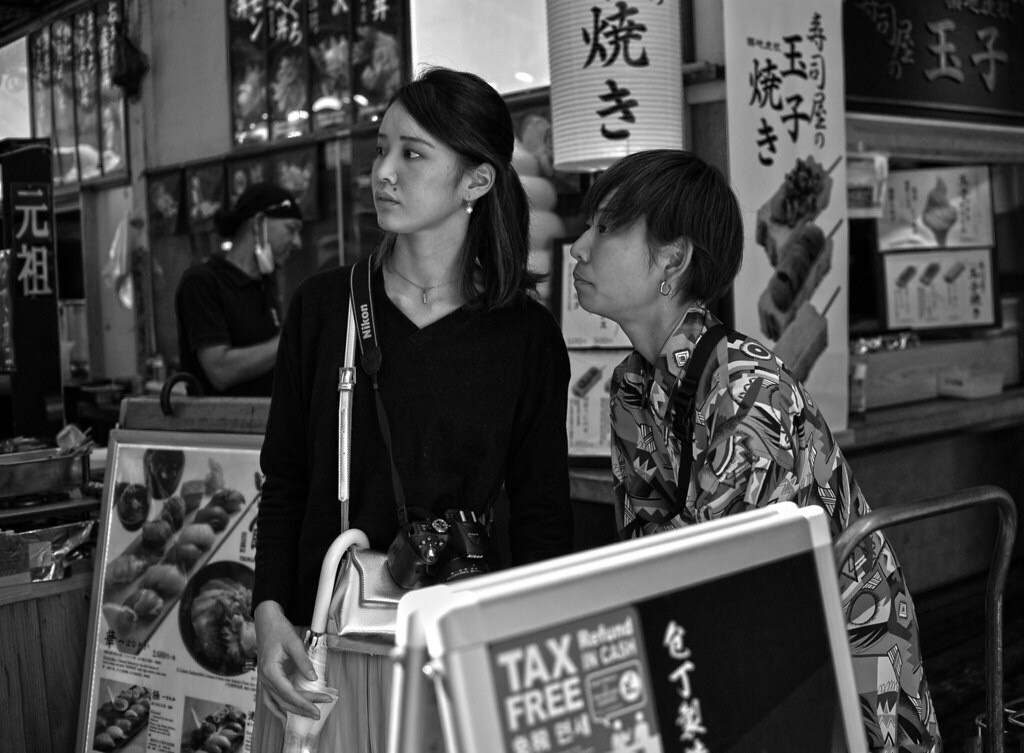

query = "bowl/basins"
[0,448,82,499]
[178,561,259,676]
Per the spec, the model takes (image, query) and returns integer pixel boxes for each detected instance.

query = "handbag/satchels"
[325,552,423,656]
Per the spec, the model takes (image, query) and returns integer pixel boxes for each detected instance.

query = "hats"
[234,182,301,224]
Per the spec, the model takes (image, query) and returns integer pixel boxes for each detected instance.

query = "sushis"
[95,687,150,749]
[189,706,246,753]
[107,477,245,621]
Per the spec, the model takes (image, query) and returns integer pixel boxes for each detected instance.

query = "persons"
[248,68,569,753]
[172,181,302,398]
[568,150,943,753]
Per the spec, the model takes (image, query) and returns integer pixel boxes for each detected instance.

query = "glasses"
[253,198,301,217]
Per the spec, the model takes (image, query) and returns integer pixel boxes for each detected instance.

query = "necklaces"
[393,269,451,304]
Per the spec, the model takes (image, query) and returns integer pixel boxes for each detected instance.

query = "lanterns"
[546,0,684,172]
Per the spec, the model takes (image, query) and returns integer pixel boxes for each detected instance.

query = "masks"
[255,217,274,272]
